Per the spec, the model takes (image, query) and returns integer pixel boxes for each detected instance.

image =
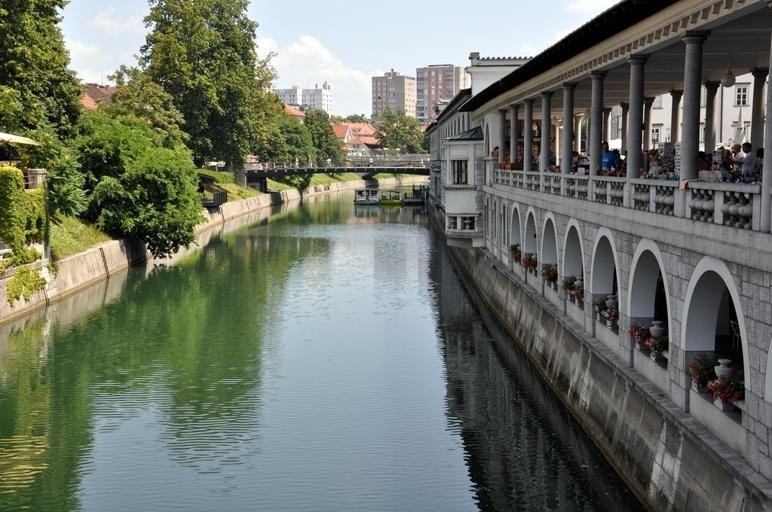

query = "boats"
[352,183,427,206]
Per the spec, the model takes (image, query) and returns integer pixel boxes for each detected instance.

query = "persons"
[490,141,765,183]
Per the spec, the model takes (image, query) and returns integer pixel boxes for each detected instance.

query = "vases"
[691,377,710,393]
[597,313,617,330]
[713,396,735,412]
[634,342,664,362]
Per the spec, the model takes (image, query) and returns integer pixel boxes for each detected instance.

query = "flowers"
[562,273,584,302]
[593,295,618,322]
[543,263,557,283]
[688,353,719,381]
[708,375,745,402]
[522,252,537,268]
[630,324,669,352]
